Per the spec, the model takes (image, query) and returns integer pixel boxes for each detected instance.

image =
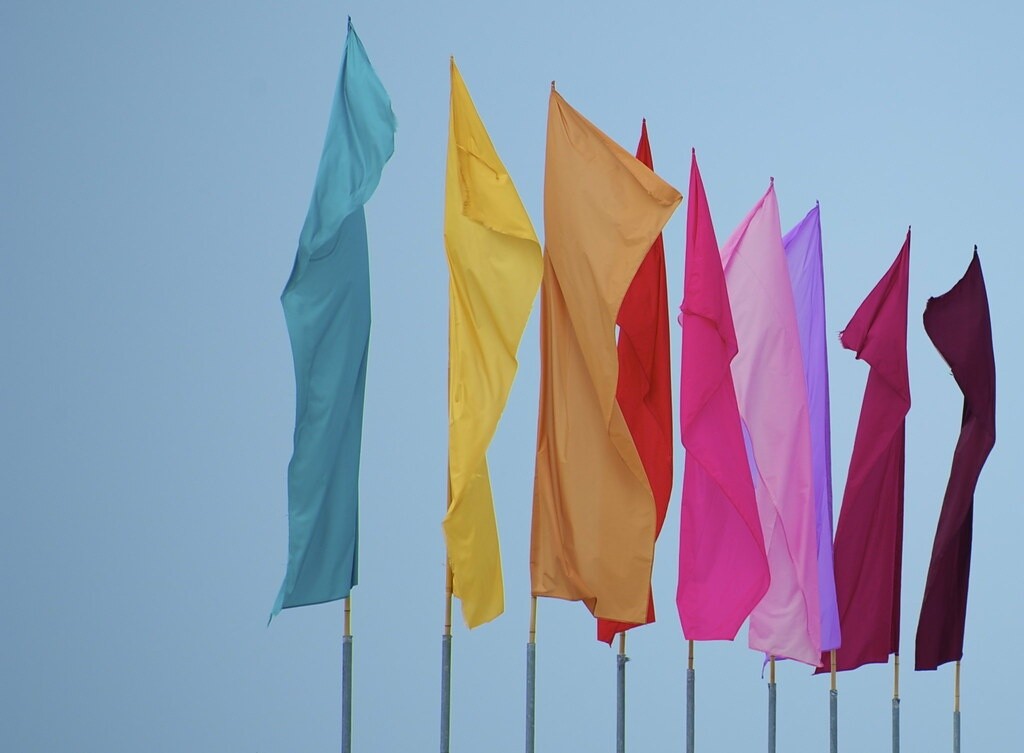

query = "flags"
[740,206,842,678]
[914,250,996,671]
[532,90,682,624]
[596,120,672,648]
[678,182,823,667]
[266,19,397,624]
[444,60,544,630]
[812,231,911,675]
[677,149,770,642]
